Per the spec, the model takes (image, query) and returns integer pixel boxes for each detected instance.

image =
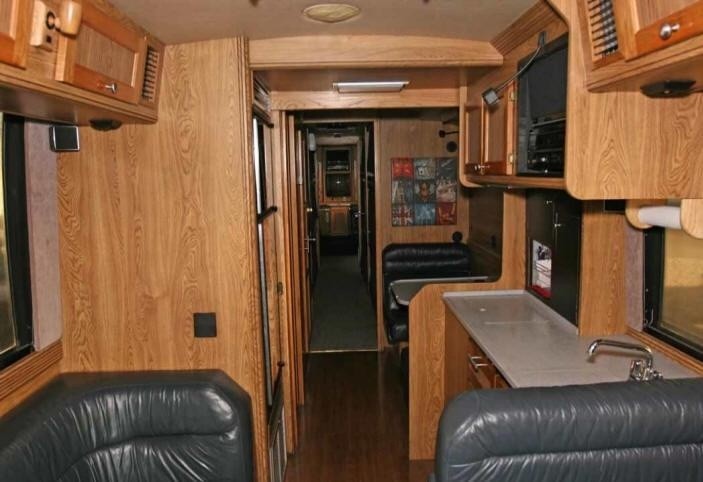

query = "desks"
[392,276,489,306]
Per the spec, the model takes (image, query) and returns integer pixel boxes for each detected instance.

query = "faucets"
[583,337,661,381]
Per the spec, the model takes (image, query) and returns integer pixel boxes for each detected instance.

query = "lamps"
[29,0,83,51]
[482,31,547,106]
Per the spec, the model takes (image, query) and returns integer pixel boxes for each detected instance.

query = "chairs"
[0,368,253,482]
[381,242,484,365]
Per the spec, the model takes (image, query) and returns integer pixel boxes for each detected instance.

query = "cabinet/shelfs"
[577,0,703,93]
[55,0,148,126]
[464,51,513,189]
[318,203,349,237]
[323,137,353,202]
[444,303,511,409]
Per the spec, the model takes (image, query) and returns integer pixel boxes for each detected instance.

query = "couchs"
[428,377,703,482]
